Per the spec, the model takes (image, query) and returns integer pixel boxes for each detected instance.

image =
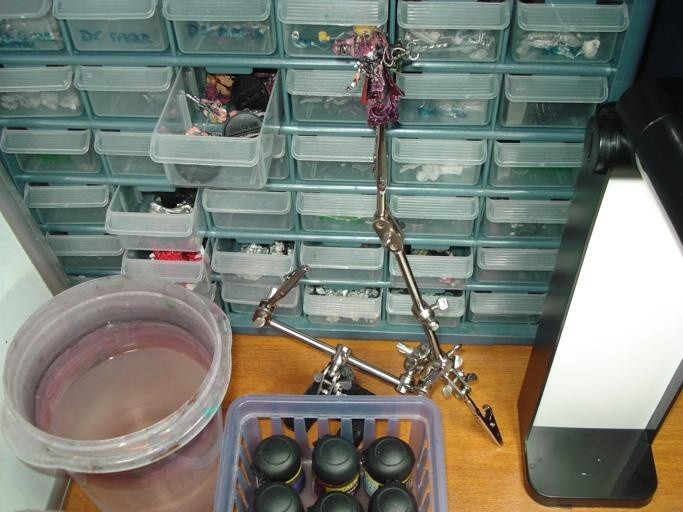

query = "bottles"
[251,435,417,511]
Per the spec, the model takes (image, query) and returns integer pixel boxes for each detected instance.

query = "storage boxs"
[0,1,631,336]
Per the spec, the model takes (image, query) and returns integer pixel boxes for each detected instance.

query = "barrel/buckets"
[0,275,232,512]
